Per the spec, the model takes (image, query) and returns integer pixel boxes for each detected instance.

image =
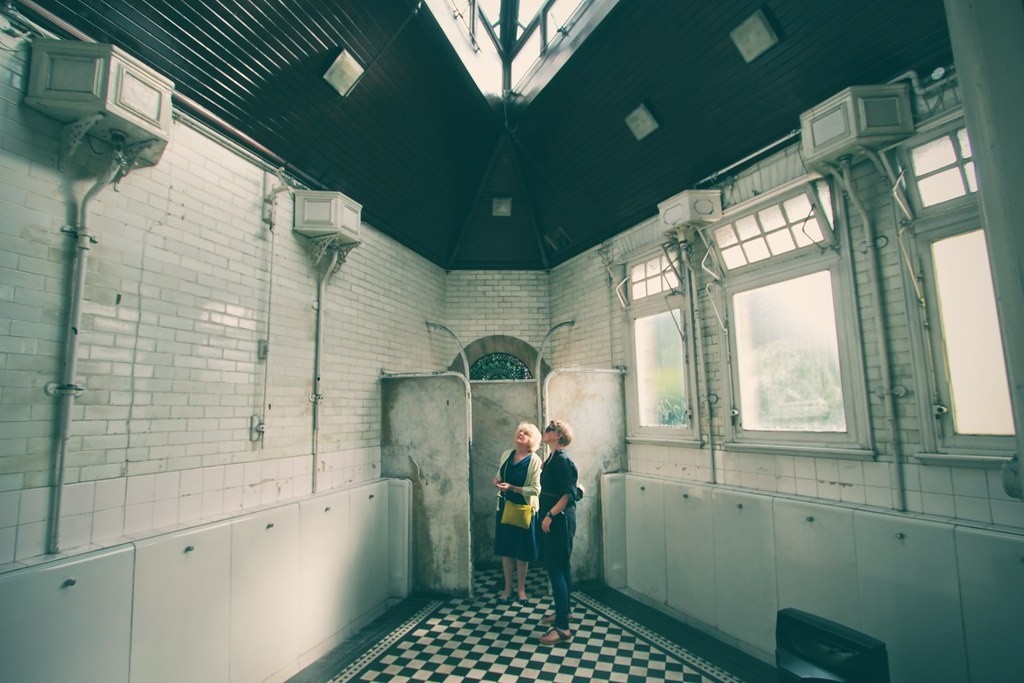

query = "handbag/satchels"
[501,499,532,529]
[575,484,585,501]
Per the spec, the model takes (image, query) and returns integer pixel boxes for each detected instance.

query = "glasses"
[546,426,555,433]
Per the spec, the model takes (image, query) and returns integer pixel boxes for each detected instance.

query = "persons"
[492,421,542,605]
[535,421,578,644]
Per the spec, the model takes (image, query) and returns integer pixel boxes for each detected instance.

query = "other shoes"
[497,593,513,604]
[514,592,529,606]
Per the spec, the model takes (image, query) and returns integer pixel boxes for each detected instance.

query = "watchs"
[547,511,554,518]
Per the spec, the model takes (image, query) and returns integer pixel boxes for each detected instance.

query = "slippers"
[540,627,571,643]
[542,613,574,623]
